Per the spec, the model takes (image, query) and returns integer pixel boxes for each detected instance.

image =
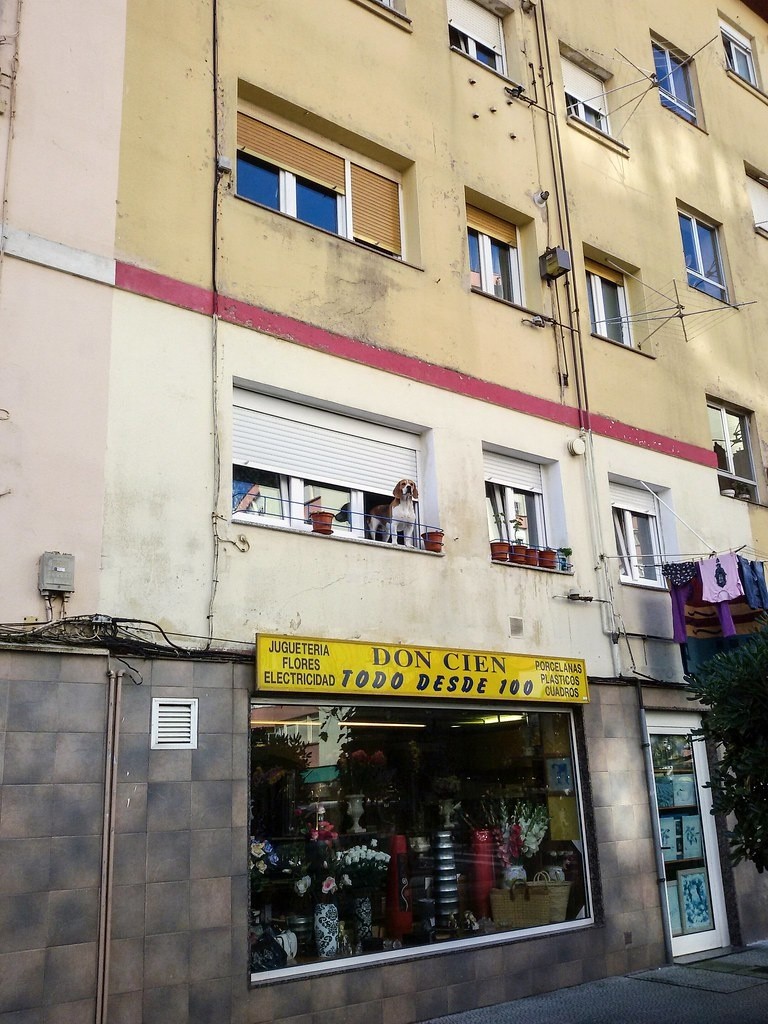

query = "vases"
[285,916,314,963]
[310,511,336,535]
[498,864,527,890]
[313,903,339,959]
[421,532,444,552]
[544,865,565,881]
[437,798,456,830]
[525,549,558,569]
[352,897,372,956]
[343,794,367,833]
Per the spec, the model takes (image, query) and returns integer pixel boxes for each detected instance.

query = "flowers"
[345,750,386,794]
[481,796,554,869]
[249,807,391,914]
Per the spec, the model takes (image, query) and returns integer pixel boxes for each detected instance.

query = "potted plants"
[509,517,527,564]
[490,513,510,561]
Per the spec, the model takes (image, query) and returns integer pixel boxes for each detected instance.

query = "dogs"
[365,479,419,548]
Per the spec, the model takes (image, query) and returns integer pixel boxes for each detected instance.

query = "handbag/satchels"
[515,871,572,922]
[490,878,551,928]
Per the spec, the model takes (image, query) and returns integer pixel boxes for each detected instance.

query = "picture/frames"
[666,867,714,937]
[543,752,575,797]
